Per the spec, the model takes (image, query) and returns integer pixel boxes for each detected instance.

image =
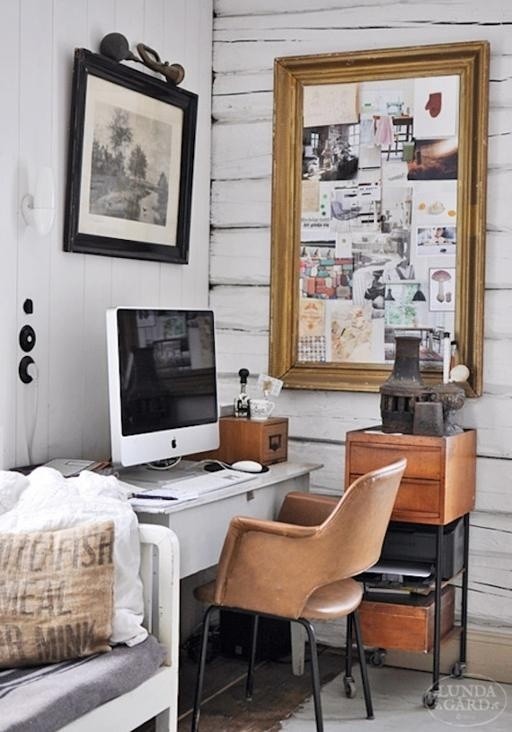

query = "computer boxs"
[219,608,292,663]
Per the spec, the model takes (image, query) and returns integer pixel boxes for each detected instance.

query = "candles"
[443,336,450,383]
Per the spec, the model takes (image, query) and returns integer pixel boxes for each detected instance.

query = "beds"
[0,467,181,731]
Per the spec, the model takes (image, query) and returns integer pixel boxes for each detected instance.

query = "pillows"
[0,523,114,666]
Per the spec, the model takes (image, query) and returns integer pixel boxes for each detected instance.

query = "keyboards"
[128,468,258,507]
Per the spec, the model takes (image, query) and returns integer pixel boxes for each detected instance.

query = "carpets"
[179,624,362,732]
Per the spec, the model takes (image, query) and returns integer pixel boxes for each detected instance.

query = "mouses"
[231,459,270,473]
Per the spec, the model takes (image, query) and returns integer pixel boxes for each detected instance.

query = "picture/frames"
[265,41,491,398]
[63,48,199,264]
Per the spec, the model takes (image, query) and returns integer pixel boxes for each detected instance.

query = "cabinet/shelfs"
[346,428,477,708]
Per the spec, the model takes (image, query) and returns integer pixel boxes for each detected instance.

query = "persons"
[417,227,456,249]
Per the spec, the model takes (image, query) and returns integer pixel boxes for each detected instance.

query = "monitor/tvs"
[105,304,221,483]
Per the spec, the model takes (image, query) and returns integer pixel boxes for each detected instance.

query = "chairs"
[190,461,409,732]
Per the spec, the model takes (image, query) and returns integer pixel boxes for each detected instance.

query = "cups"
[250,398,275,421]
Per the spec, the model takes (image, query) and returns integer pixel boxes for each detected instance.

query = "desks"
[61,455,323,732]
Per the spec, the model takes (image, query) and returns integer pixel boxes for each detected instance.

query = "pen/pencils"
[133,494,178,500]
